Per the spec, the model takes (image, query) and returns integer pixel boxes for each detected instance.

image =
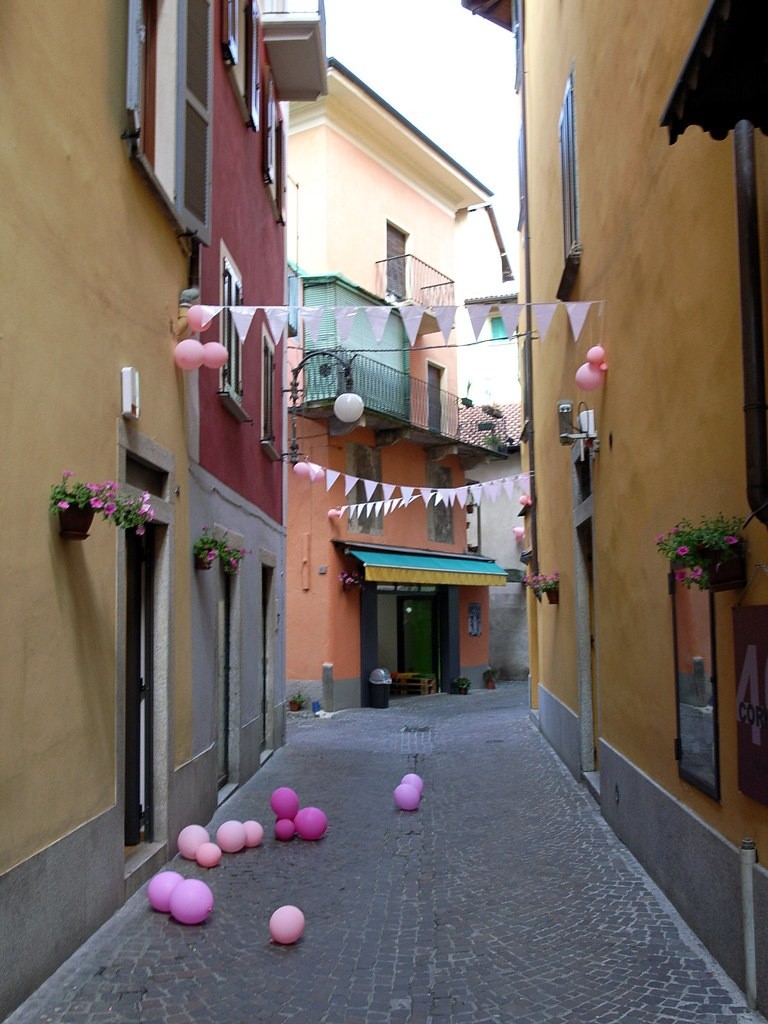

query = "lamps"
[282,348,365,471]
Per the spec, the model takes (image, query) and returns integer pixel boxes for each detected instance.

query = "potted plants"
[482,666,497,690]
[289,692,306,710]
[482,434,502,451]
[454,674,471,695]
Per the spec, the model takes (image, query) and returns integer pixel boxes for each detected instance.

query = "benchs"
[392,672,437,696]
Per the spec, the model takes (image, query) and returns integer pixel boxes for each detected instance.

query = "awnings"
[347,548,508,586]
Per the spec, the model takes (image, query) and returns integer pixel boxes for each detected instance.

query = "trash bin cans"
[368,668,392,708]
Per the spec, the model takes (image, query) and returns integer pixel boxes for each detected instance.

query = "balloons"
[186,305,211,331]
[274,819,295,840]
[147,872,184,913]
[217,820,246,853]
[177,824,209,860]
[293,462,325,482]
[401,774,423,794]
[576,346,607,391]
[520,496,532,504]
[328,509,341,519]
[169,879,214,924]
[271,788,298,819]
[294,807,327,838]
[513,528,525,541]
[269,905,304,944]
[196,842,221,868]
[395,784,420,810]
[174,339,227,369]
[243,821,264,847]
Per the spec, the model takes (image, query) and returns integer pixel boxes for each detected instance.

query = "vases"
[59,502,96,540]
[653,510,750,593]
[545,589,560,604]
[697,537,747,592]
[344,583,356,593]
[195,554,212,571]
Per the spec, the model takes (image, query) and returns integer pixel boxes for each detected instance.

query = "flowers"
[193,523,254,576]
[523,570,560,594]
[336,567,365,592]
[48,468,156,535]
